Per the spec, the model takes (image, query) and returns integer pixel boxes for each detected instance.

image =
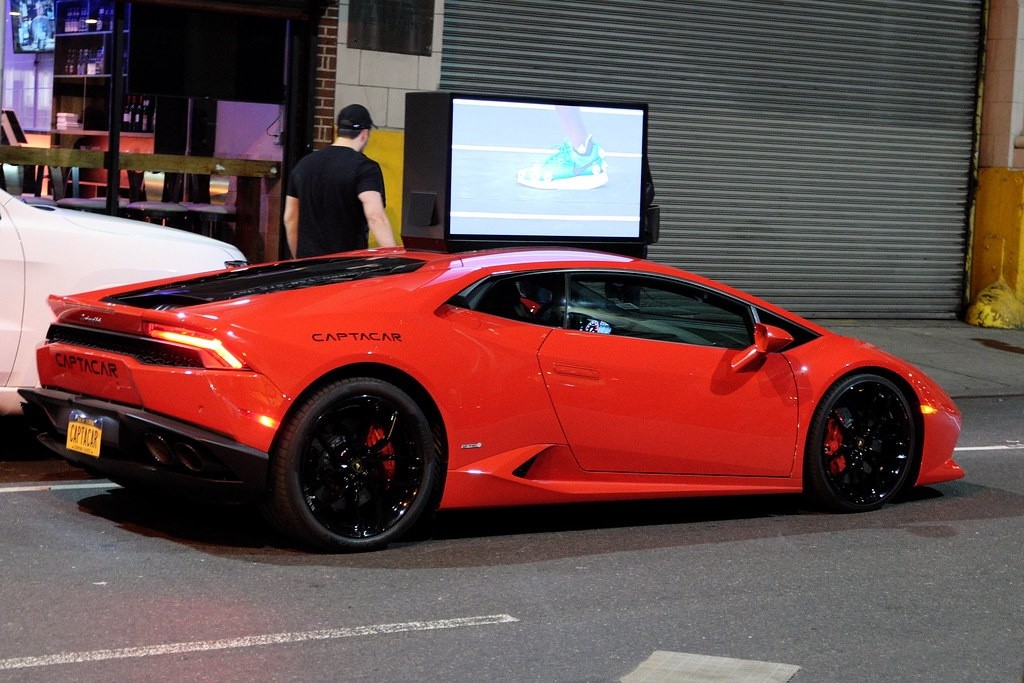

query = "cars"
[0,188,252,419]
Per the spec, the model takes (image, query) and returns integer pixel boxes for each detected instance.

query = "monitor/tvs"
[451,98,643,237]
[10,0,56,53]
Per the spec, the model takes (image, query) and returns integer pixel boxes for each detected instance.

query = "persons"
[283,104,397,259]
[19,1,52,47]
[516,106,608,190]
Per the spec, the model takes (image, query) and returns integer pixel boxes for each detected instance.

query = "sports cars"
[16,244,967,551]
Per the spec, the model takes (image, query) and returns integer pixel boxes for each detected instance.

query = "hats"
[338,104,379,130]
